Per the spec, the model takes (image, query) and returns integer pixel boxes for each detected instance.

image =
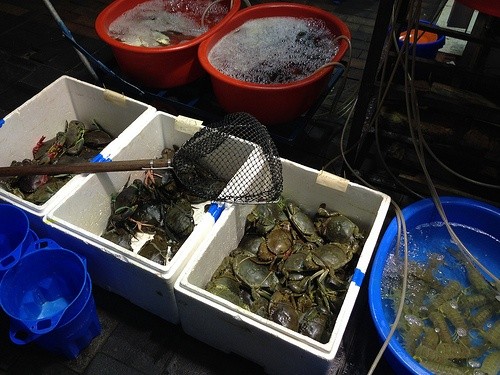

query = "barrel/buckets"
[0,201,105,363]
[385,19,448,62]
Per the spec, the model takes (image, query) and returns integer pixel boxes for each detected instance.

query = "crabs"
[100,144,228,266]
[205,189,368,345]
[0,118,115,206]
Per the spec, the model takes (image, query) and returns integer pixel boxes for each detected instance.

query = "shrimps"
[382,246,500,375]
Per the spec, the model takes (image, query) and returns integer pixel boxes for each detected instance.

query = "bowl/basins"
[370,195,500,375]
[196,3,353,122]
[93,1,241,90]
